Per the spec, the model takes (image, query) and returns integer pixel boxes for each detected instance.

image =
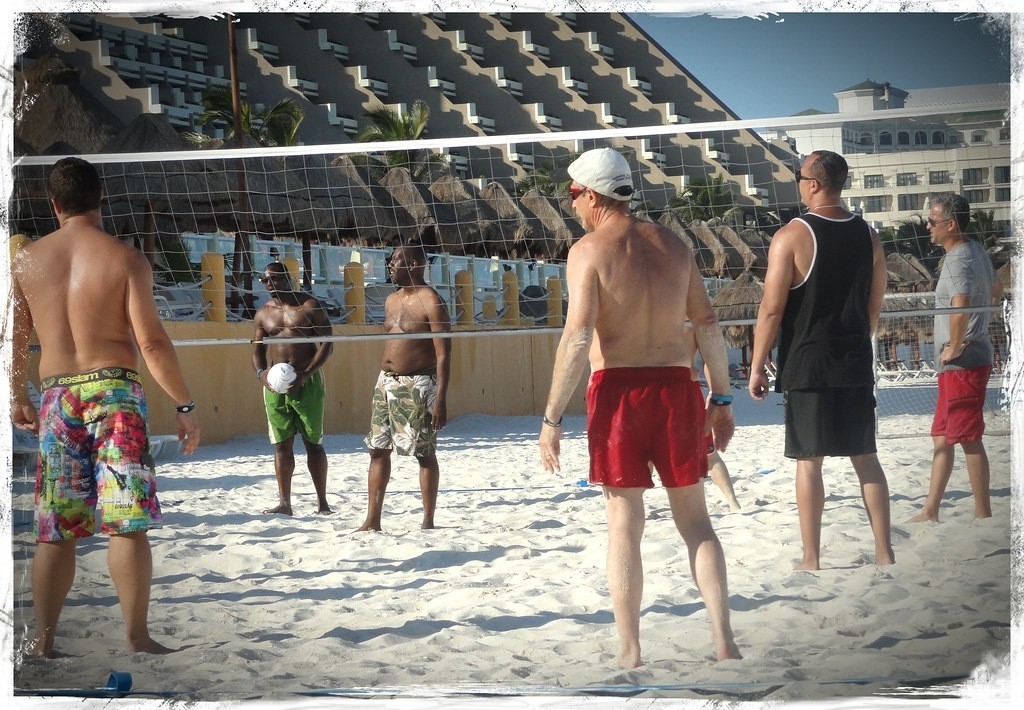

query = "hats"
[568,148,634,202]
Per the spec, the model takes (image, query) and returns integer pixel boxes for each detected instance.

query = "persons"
[251,262,333,516]
[14,156,202,659]
[352,242,452,533]
[537,147,741,672]
[748,150,896,570]
[903,192,1005,524]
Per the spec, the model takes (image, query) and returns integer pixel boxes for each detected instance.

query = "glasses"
[795,170,819,183]
[927,216,954,227]
[261,275,284,285]
[568,187,588,200]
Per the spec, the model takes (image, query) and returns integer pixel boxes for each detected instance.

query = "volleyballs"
[266,362,298,394]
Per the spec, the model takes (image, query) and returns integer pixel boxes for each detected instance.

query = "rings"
[545,454,549,455]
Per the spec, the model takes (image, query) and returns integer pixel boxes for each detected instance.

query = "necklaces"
[810,204,843,212]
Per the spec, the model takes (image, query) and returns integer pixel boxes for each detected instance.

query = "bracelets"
[710,393,733,407]
[175,401,195,413]
[543,413,562,427]
[256,368,264,376]
